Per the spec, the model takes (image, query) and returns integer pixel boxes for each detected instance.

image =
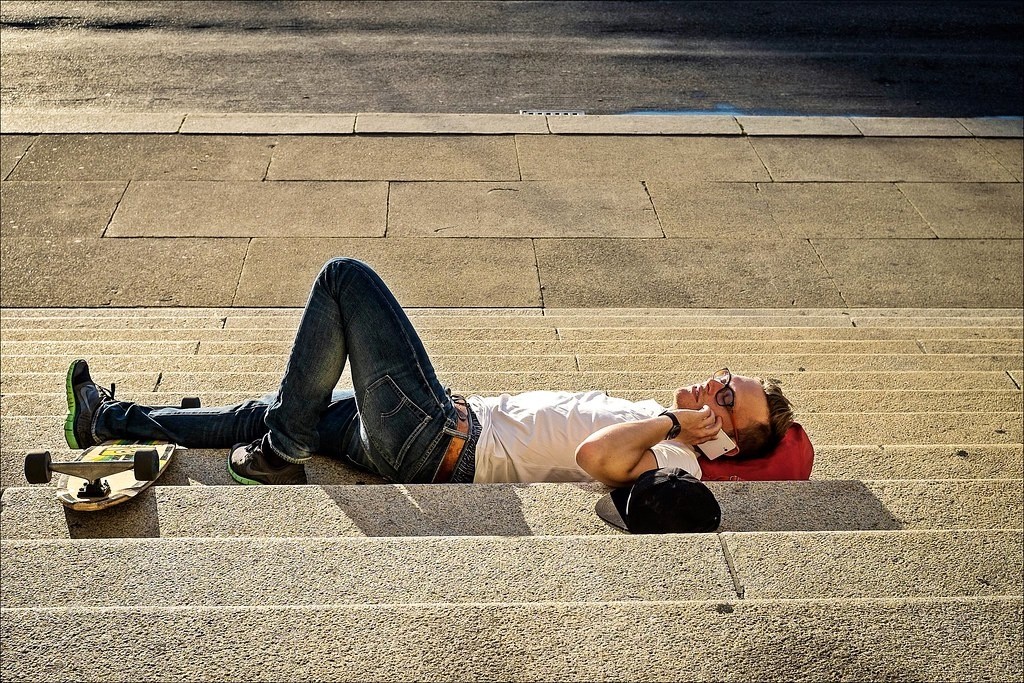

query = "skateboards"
[24,396,202,511]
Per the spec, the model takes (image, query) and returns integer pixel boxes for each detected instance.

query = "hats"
[595,467,721,533]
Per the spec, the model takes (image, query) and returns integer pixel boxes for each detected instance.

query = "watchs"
[658,410,681,440]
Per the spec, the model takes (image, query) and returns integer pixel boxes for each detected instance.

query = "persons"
[64,258,791,485]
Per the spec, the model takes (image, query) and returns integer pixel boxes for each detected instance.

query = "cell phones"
[697,417,737,460]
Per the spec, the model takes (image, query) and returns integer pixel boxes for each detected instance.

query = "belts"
[441,395,469,481]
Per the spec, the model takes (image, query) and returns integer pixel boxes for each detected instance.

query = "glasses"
[713,367,739,444]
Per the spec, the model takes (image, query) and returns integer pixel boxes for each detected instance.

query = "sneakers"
[65,359,115,450]
[227,430,312,485]
